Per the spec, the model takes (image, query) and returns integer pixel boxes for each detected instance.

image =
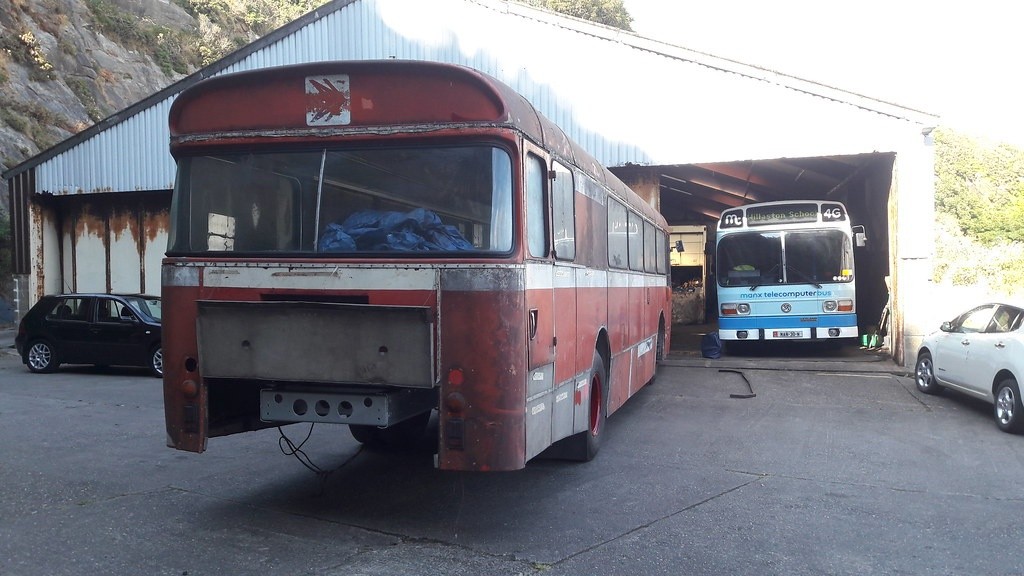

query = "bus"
[159,58,685,471]
[704,200,867,344]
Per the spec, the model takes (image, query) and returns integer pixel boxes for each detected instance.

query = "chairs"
[56,305,73,319]
[98,307,111,321]
[120,307,135,321]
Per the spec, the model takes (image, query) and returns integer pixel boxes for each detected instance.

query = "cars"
[15,293,162,379]
[914,298,1024,431]
